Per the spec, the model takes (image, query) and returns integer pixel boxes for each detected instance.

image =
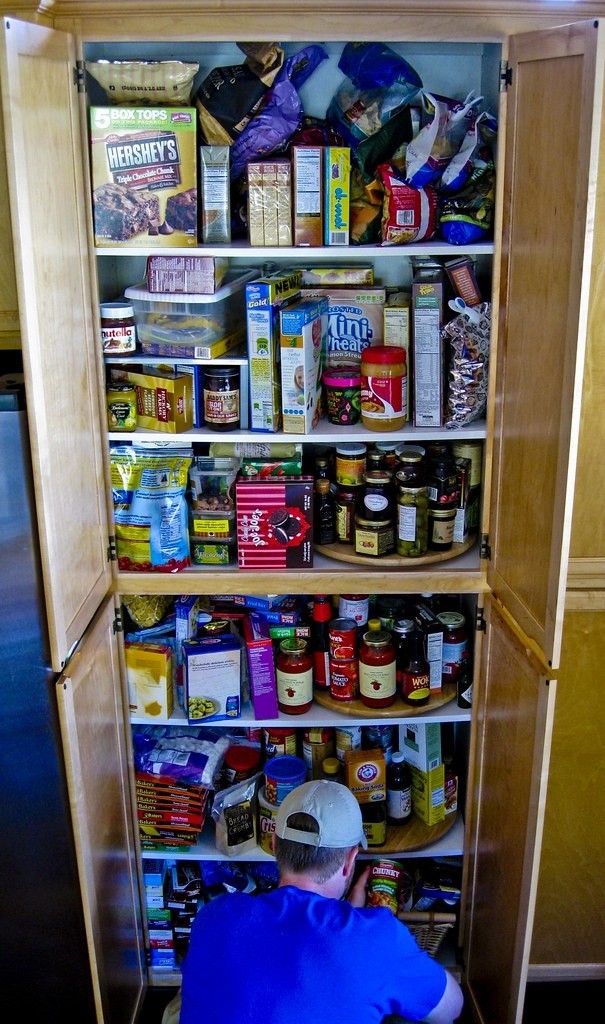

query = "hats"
[275,780,367,850]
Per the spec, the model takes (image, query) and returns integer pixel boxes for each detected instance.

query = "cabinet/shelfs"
[0,0,605,1024]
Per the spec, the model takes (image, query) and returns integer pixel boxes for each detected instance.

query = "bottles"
[404,631,432,705]
[387,751,413,822]
[321,759,345,785]
[456,652,473,708]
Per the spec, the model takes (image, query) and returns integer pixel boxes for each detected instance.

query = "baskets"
[402,923,454,957]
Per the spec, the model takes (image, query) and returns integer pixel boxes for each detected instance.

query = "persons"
[161,779,463,1024]
